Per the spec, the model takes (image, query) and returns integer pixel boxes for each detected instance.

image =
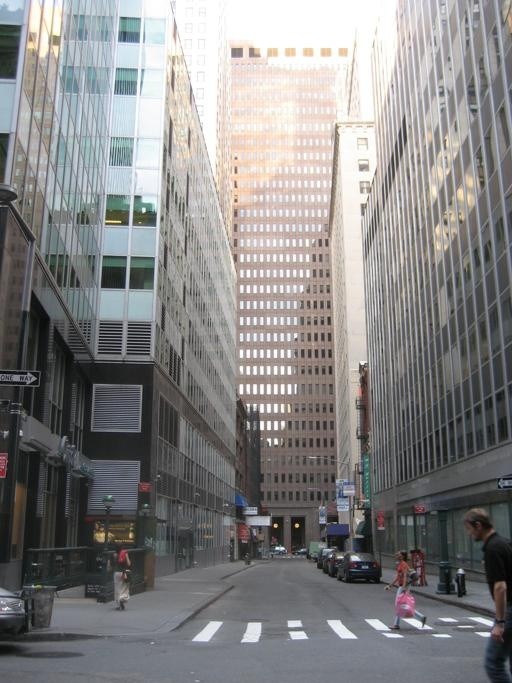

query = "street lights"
[308,455,353,550]
[98,492,117,604]
[140,502,153,548]
[0,183,38,565]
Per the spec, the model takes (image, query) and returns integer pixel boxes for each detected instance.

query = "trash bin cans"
[245,553,251,566]
[258,552,262,559]
[22,585,58,627]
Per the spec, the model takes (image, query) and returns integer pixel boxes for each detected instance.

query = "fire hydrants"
[453,567,467,598]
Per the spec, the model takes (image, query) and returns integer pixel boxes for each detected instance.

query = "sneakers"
[421,618,427,628]
[120,600,125,610]
[390,625,399,629]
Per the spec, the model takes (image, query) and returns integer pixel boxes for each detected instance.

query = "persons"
[460,507,512,683]
[106,541,132,611]
[383,549,427,629]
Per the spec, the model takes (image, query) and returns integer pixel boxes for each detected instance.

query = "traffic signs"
[1,369,41,387]
[496,476,512,490]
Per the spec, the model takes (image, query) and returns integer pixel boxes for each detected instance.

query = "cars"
[292,540,381,583]
[271,546,288,555]
[0,586,28,634]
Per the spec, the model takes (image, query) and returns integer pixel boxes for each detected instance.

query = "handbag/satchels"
[399,568,419,585]
[124,571,129,583]
